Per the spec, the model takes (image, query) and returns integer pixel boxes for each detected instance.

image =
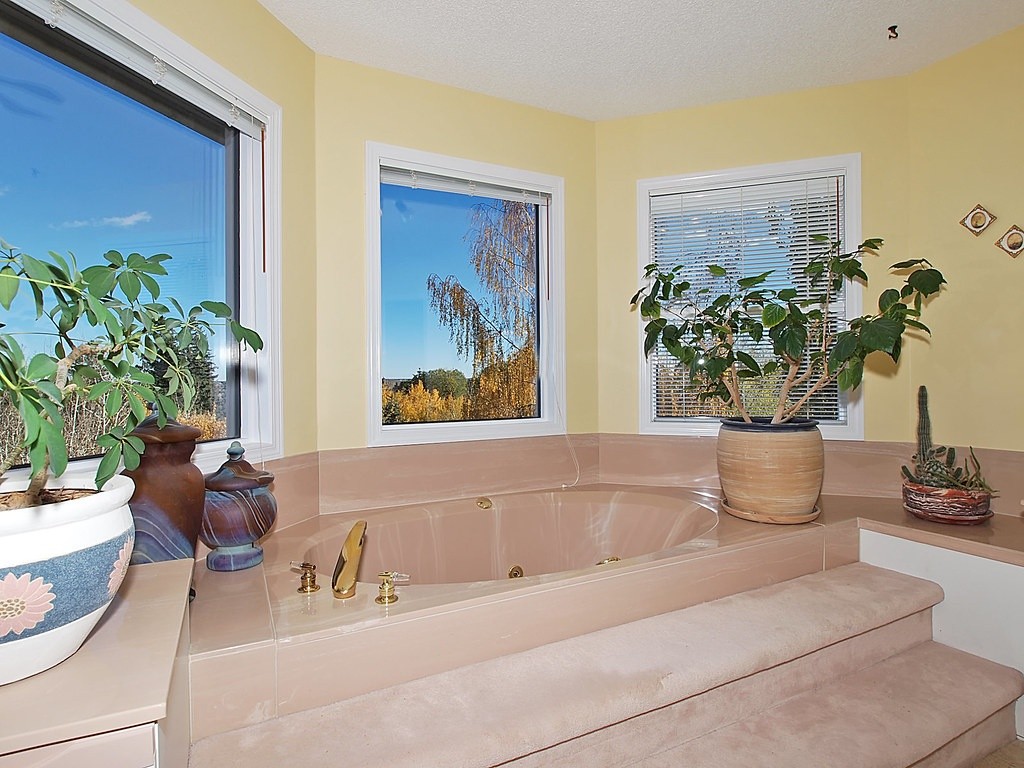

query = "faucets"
[331,519,369,600]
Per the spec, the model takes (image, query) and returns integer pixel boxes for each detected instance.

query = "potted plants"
[0,237,263,686]
[628,238,947,523]
[901,385,1001,526]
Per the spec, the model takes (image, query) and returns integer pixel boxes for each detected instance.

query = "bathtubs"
[292,489,721,586]
